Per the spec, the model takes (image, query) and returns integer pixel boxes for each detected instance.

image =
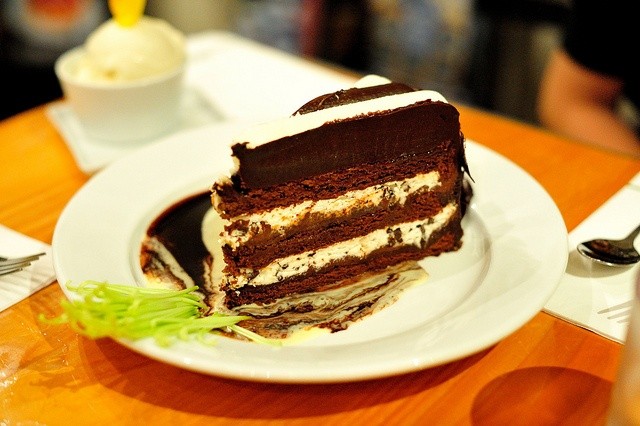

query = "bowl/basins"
[53,44,185,148]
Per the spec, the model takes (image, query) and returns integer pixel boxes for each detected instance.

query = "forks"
[0,252,46,277]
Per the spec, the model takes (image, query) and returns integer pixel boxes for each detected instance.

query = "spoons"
[577,223,640,269]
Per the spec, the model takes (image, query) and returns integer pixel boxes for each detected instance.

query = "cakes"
[140,74,475,342]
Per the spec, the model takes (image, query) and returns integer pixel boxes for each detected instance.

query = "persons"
[536,0,640,159]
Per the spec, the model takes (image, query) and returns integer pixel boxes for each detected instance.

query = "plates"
[46,82,231,176]
[50,113,572,386]
